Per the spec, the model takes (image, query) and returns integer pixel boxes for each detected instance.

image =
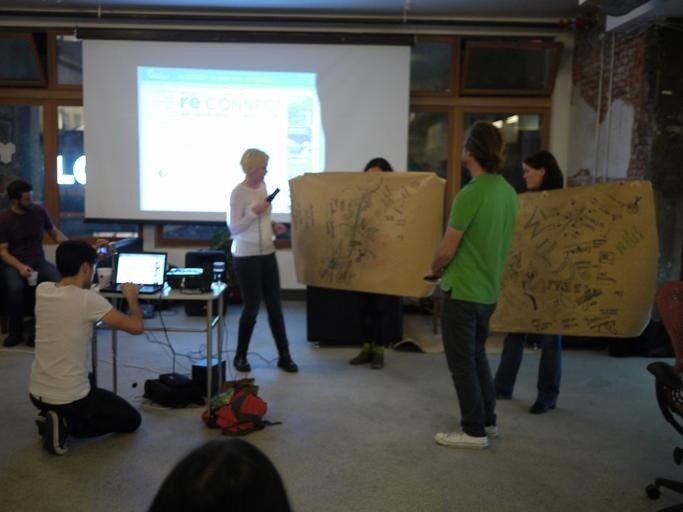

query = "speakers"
[191,357,226,398]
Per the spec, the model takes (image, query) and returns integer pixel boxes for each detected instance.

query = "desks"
[90,281,228,419]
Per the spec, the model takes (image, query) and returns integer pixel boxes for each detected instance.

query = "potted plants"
[208,231,242,304]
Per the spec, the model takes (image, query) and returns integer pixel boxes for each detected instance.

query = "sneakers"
[43,411,68,456]
[34,412,46,434]
[350,351,371,365]
[25,334,35,349]
[3,334,23,347]
[235,355,251,371]
[482,426,499,440]
[279,358,298,372]
[529,401,557,414]
[372,351,384,369]
[434,427,489,449]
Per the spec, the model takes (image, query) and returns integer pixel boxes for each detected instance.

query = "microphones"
[266,188,280,202]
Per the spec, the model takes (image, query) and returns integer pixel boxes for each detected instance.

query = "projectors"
[166,267,204,290]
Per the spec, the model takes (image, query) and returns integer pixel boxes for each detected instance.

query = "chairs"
[644,280,683,511]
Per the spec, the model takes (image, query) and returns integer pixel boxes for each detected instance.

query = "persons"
[147,439,291,512]
[0,180,69,348]
[27,241,144,456]
[494,151,564,415]
[423,121,518,449]
[224,150,298,373]
[349,157,394,368]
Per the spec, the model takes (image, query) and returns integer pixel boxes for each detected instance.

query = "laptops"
[99,251,167,294]
